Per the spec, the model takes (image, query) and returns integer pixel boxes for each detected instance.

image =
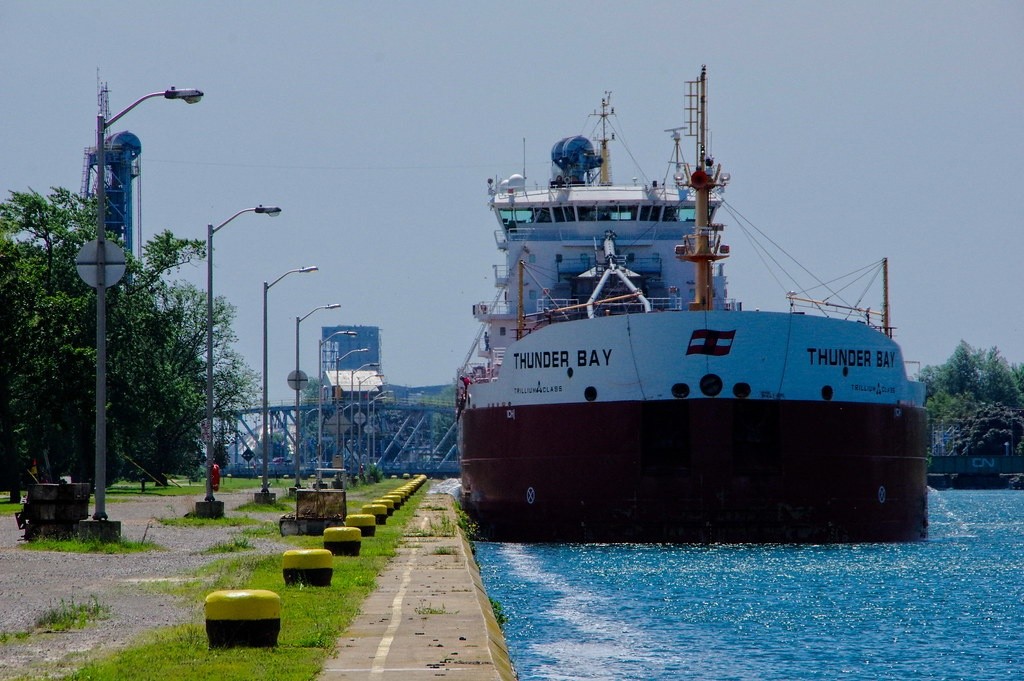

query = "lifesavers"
[211,464,220,492]
[473,366,486,380]
[360,466,363,476]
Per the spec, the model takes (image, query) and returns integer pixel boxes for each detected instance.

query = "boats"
[457,63,931,544]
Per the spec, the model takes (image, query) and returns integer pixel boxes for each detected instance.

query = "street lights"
[331,347,369,488]
[372,389,393,468]
[290,302,342,496]
[255,265,319,502]
[357,374,384,485]
[336,402,359,469]
[346,361,380,486]
[303,408,319,474]
[313,328,357,488]
[196,203,282,519]
[77,89,205,542]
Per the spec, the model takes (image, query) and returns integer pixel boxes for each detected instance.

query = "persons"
[456,376,472,411]
[484,332,489,351]
[551,175,566,188]
